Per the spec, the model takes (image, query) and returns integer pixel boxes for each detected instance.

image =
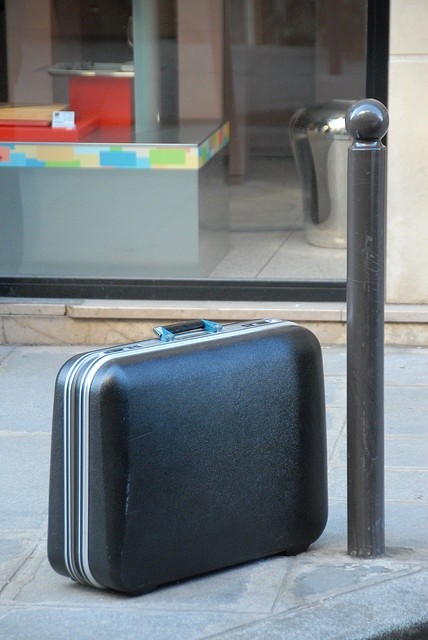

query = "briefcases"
[50,321,326,596]
